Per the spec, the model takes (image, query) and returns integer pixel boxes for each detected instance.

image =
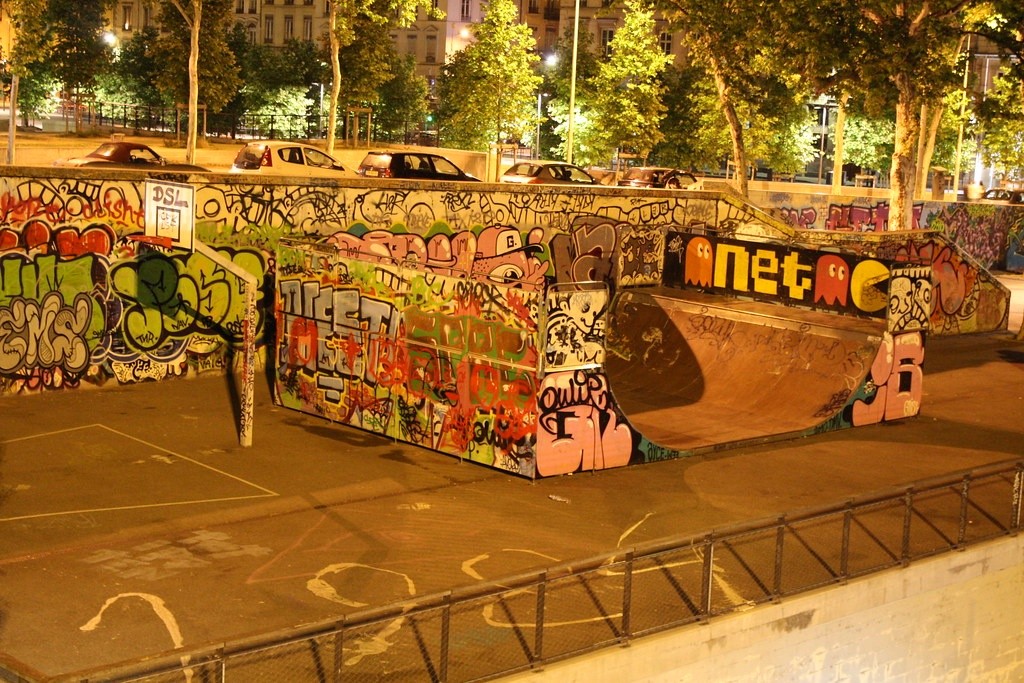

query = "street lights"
[534,55,560,159]
[319,62,328,138]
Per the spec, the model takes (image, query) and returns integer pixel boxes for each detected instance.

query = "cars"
[974,187,1024,205]
[617,167,697,189]
[500,160,605,185]
[63,139,213,173]
[228,141,362,179]
[355,151,483,181]
